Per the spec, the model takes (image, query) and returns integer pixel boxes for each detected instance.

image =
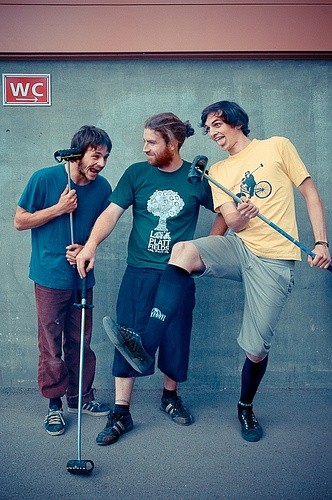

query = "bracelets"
[315,240,328,247]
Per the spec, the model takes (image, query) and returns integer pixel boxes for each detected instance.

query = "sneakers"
[160,396,193,425]
[95,410,133,445]
[44,406,65,435]
[238,401,263,442]
[103,315,154,374]
[67,399,110,416]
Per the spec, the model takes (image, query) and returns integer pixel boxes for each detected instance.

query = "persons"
[76,111,228,445]
[13,125,113,436]
[105,101,331,442]
